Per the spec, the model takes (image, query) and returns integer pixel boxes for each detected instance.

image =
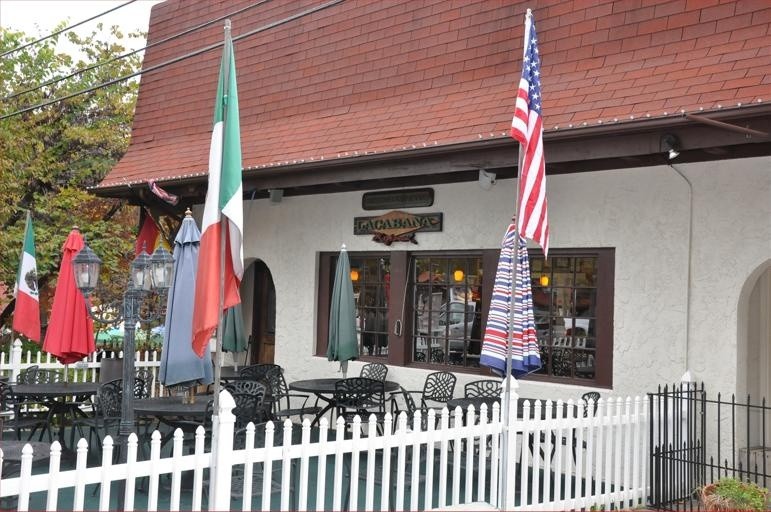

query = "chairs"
[0,363,267,497]
[240,363,457,461]
[399,379,600,495]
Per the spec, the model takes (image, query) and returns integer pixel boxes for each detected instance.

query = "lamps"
[662,136,682,161]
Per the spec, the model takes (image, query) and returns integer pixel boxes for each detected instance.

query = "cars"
[351,293,591,365]
[0,307,168,345]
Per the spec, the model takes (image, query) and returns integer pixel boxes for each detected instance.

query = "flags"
[137,208,171,256]
[510,8,550,262]
[192,19,246,358]
[12,210,42,347]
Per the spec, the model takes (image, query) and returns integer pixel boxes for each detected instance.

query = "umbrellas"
[160,208,214,403]
[480,215,544,414]
[324,244,361,381]
[43,228,97,385]
[222,304,246,371]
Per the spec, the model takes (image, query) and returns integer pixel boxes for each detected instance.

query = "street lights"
[73,233,181,438]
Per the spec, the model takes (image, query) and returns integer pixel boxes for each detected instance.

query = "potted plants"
[689,477,771,512]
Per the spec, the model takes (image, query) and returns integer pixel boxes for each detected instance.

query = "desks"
[130,395,214,442]
[445,393,583,422]
[1,382,104,453]
[288,377,399,440]
